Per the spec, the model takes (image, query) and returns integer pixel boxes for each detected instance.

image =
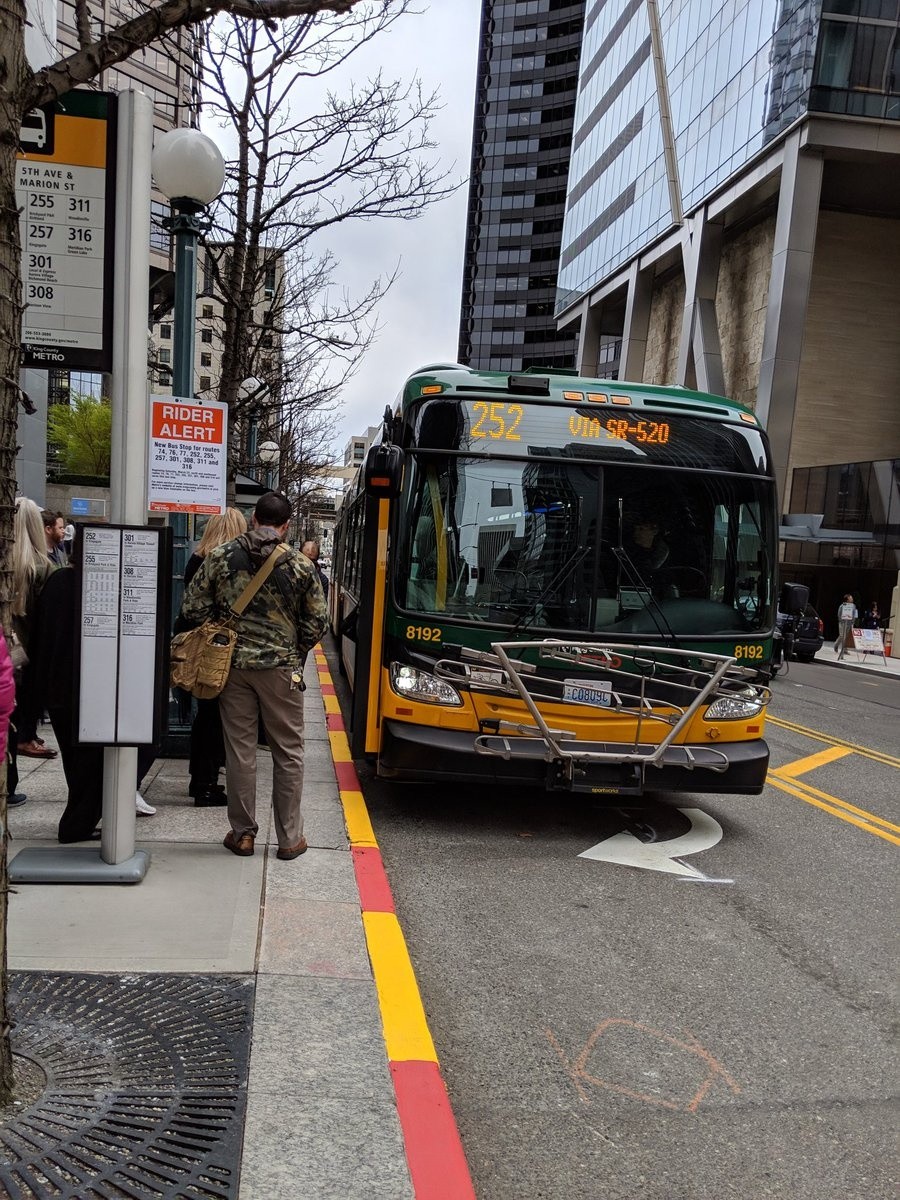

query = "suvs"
[774,582,825,662]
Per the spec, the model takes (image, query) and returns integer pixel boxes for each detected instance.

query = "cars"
[317,538,334,569]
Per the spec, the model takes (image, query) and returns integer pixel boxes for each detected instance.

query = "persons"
[183,506,247,808]
[624,515,691,585]
[181,492,328,860]
[835,594,857,655]
[0,497,158,843]
[863,602,880,655]
[299,541,328,605]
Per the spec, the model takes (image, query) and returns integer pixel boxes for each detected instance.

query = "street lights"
[258,438,280,493]
[234,375,271,484]
[152,129,223,758]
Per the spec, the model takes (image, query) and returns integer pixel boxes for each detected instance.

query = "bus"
[326,365,809,794]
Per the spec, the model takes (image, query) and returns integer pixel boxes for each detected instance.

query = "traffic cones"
[884,636,892,657]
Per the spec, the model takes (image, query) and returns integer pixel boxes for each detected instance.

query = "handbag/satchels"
[169,618,239,700]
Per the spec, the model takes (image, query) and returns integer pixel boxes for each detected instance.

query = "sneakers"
[6,793,26,807]
[135,791,156,815]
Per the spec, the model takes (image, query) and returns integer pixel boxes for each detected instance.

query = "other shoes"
[58,820,102,842]
[257,734,273,751]
[195,782,228,807]
[189,779,225,797]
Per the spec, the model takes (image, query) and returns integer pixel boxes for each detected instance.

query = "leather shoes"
[223,829,254,856]
[35,739,44,745]
[277,836,307,860]
[16,739,57,757]
